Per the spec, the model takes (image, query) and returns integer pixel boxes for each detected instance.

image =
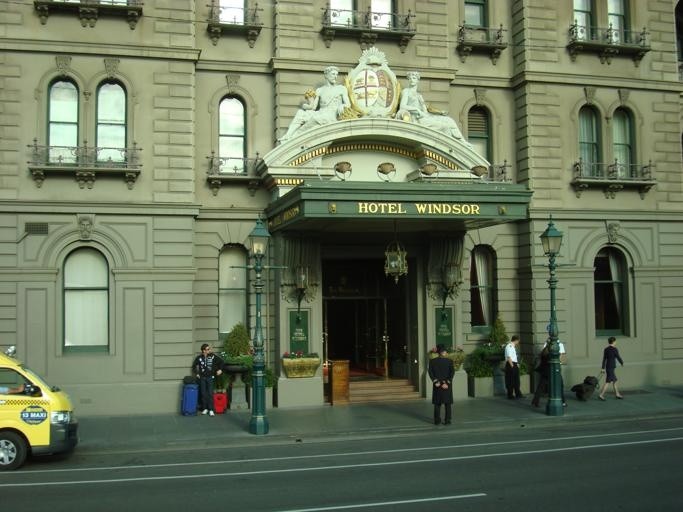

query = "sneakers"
[506,395,525,400]
[201,409,214,417]
[531,399,541,408]
[597,394,624,401]
[562,402,568,407]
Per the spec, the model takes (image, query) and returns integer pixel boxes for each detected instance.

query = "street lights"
[539,212,565,416]
[249,218,270,435]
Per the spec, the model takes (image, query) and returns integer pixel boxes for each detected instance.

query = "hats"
[434,344,448,353]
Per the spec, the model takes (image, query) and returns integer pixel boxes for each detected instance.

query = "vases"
[283,359,321,377]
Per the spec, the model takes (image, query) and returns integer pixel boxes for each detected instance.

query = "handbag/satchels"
[182,374,200,384]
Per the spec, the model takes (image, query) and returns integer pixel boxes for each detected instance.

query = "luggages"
[212,374,227,414]
[571,371,604,402]
[181,384,199,416]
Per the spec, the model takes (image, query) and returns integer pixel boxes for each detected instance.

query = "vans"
[0,351,78,475]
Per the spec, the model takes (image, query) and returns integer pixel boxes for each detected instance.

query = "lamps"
[444,262,464,294]
[294,265,312,292]
[384,219,408,288]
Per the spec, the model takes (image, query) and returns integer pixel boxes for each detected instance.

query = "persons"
[598,336,624,401]
[531,329,568,408]
[428,343,454,425]
[504,335,526,400]
[276,67,350,143]
[0,382,24,394]
[399,72,472,149]
[192,344,224,417]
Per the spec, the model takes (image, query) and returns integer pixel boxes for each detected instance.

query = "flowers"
[282,350,319,360]
[430,345,463,355]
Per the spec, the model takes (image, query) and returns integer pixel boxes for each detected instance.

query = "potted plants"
[219,321,276,412]
